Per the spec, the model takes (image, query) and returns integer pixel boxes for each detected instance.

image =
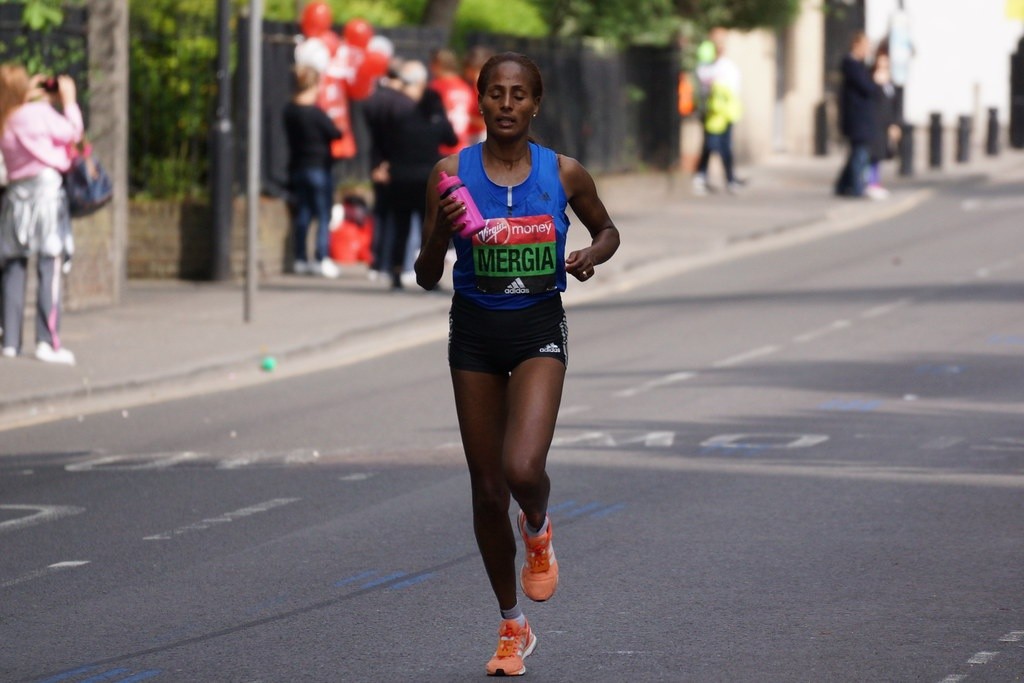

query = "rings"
[582,270,587,278]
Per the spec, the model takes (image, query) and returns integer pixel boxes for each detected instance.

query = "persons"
[833,29,897,198]
[0,64,84,363]
[361,49,487,288]
[414,54,620,676]
[283,67,343,279]
[665,22,751,194]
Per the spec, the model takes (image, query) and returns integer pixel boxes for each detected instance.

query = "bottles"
[436,170,485,238]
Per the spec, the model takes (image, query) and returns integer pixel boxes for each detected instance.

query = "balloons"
[301,0,392,98]
[333,195,375,264]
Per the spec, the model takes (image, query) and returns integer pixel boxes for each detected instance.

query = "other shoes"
[835,183,858,198]
[860,181,889,200]
[390,273,402,288]
[295,257,341,280]
[367,268,381,283]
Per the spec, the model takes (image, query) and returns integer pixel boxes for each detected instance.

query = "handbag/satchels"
[63,155,112,218]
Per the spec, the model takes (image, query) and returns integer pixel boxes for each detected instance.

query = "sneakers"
[35,341,74,364]
[1,345,18,358]
[517,508,561,601]
[486,618,536,675]
